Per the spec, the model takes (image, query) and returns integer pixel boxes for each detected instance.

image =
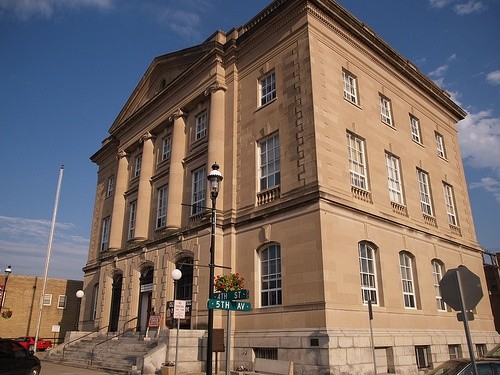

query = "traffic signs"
[207,289,251,313]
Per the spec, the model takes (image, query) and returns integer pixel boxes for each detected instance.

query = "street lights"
[170,269,182,328]
[0,264,11,313]
[75,287,85,330]
[205,161,223,375]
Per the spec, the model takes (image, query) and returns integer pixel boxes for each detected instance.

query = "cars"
[423,358,499,375]
[0,339,43,375]
[13,337,52,352]
